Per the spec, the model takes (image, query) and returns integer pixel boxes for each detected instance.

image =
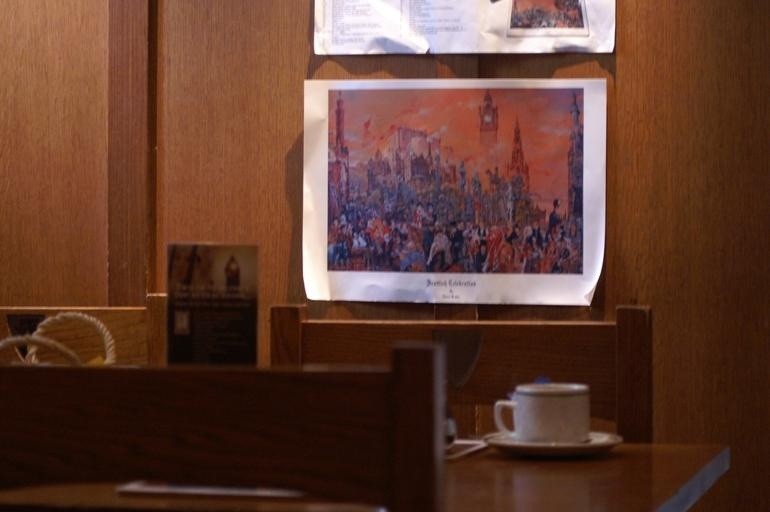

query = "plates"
[481,431,624,458]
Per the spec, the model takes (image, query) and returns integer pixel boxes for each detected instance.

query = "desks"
[2,431,732,511]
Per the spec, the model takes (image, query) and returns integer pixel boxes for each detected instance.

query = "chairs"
[270,305,654,441]
[1,292,169,368]
[1,341,447,511]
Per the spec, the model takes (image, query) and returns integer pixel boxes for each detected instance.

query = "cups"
[492,381,590,444]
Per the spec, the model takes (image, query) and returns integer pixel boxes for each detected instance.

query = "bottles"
[223,251,242,292]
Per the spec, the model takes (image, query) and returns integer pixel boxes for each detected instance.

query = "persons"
[328,144,582,273]
[225,253,240,293]
